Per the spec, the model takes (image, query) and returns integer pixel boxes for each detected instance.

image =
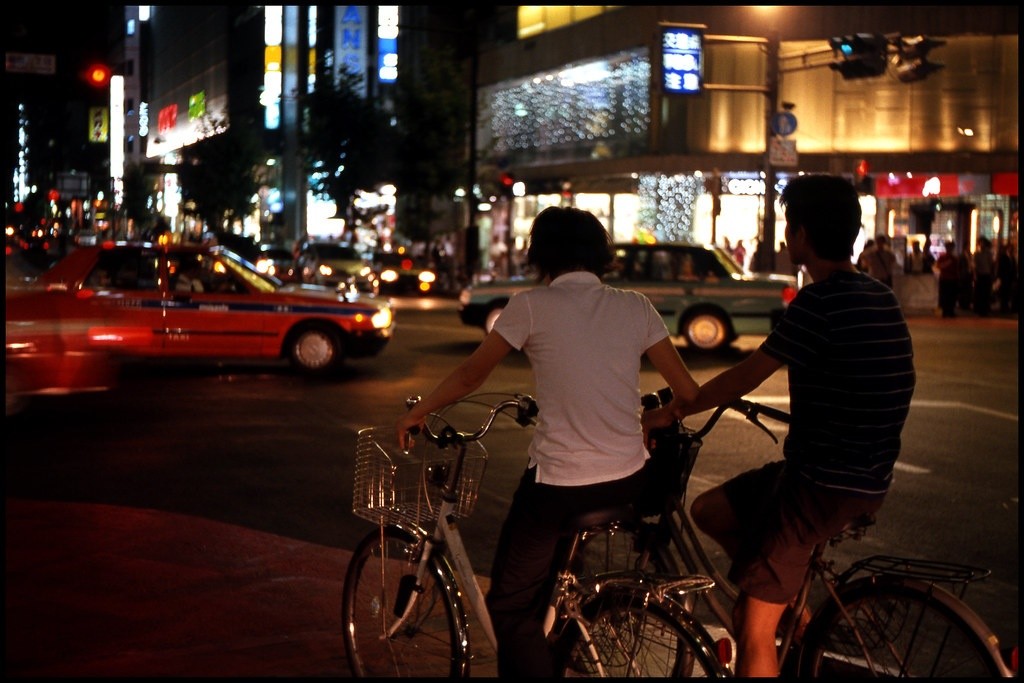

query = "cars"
[459,241,796,358]
[5,241,395,386]
[216,231,444,297]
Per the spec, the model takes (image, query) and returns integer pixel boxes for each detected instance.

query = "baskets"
[634,424,704,520]
[350,423,490,527]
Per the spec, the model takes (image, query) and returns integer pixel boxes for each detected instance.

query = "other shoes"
[943,310,947,317]
[947,311,955,317]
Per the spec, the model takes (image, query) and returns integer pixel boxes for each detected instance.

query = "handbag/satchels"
[887,273,893,288]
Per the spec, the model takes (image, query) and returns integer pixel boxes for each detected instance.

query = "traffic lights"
[826,33,887,79]
[895,34,947,83]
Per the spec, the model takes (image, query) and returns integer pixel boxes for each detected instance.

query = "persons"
[639,173,917,677]
[722,238,793,276]
[854,231,1024,320]
[430,230,532,296]
[397,206,701,680]
[92,255,232,293]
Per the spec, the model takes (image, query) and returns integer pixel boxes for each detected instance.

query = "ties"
[190,284,194,293]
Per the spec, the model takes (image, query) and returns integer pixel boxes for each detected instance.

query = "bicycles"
[639,382,1024,683]
[338,395,729,683]
